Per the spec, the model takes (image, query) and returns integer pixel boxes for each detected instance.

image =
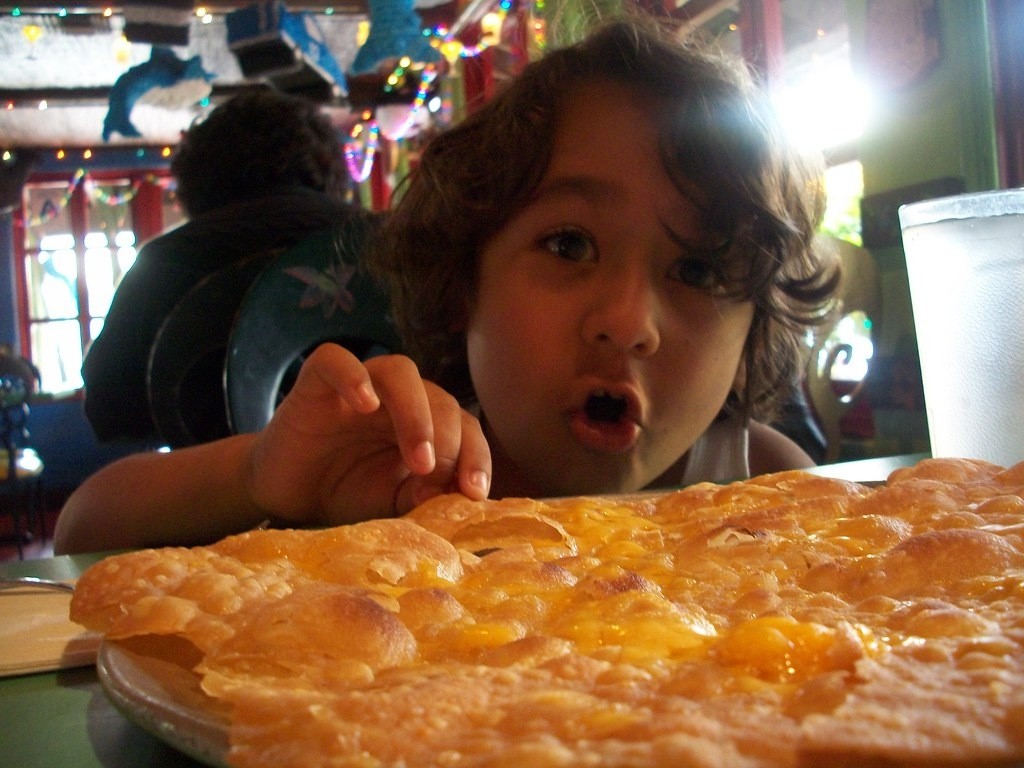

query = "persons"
[53,0,819,558]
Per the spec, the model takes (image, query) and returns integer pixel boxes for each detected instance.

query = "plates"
[97,638,231,768]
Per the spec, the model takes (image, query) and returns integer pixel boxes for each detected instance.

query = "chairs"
[146,226,449,451]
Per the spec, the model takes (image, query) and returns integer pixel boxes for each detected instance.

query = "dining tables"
[0,447,1024,768]
